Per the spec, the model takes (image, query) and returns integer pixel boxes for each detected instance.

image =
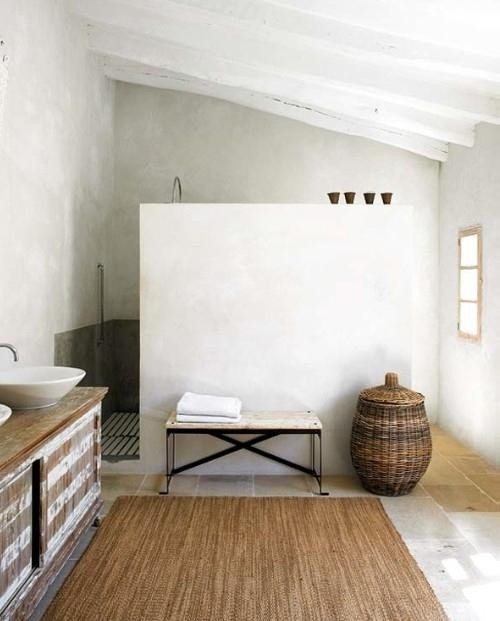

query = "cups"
[381,193,393,204]
[363,193,377,204]
[328,193,340,204]
[343,192,355,204]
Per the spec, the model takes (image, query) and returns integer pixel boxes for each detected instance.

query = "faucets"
[0,343,25,366]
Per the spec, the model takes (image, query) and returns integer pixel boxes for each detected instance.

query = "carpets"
[40,496,451,620]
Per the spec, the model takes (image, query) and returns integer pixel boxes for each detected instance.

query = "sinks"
[0,365,86,412]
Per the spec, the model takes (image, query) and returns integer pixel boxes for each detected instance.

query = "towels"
[176,391,242,417]
[177,415,240,424]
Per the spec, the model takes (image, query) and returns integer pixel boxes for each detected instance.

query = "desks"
[158,411,329,497]
